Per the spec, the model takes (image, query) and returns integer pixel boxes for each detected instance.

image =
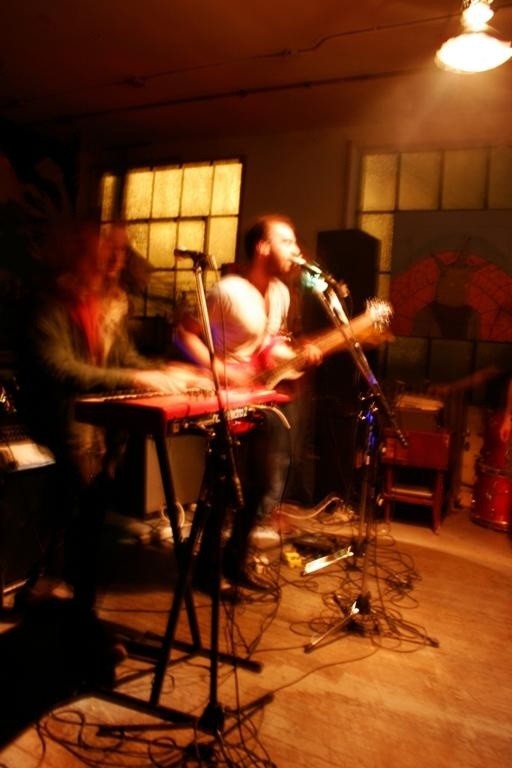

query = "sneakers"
[193,560,269,601]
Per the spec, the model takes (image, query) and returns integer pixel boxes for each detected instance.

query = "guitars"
[220,299,393,432]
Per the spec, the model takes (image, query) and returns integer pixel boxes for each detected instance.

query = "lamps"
[434,0,512,76]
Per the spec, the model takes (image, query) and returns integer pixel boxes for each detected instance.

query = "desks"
[382,426,454,535]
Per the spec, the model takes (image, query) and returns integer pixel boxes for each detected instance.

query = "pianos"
[65,387,290,432]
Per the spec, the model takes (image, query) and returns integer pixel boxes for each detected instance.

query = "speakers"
[315,225,387,496]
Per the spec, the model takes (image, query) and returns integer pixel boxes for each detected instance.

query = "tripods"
[95,262,275,768]
[304,287,443,650]
[300,291,411,587]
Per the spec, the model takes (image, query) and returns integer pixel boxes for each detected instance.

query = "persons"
[18,200,217,666]
[170,214,327,603]
[410,258,487,344]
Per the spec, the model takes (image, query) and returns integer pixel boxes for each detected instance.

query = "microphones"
[291,256,346,298]
[172,247,206,263]
